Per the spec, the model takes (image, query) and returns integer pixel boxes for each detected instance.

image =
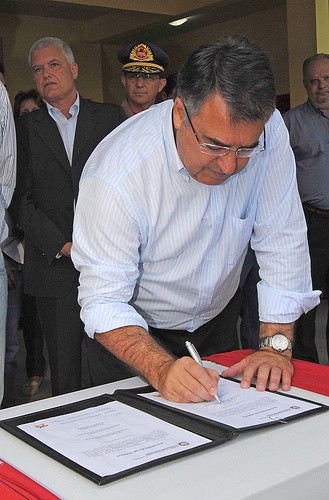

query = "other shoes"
[23,376,43,394]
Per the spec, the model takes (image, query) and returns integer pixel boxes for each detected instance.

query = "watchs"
[258,332,293,353]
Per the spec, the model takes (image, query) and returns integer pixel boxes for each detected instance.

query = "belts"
[304,203,329,216]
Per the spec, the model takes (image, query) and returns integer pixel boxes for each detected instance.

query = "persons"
[118,41,170,118]
[70,34,322,404]
[160,72,181,101]
[0,57,46,410]
[15,37,129,397]
[238,53,329,366]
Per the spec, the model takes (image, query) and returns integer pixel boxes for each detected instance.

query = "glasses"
[125,73,159,82]
[180,98,266,157]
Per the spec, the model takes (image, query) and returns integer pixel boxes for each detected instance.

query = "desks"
[0,348,329,500]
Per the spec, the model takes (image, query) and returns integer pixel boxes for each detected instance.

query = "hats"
[118,41,169,75]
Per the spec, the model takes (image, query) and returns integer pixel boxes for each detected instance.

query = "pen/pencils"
[185,339,222,405]
